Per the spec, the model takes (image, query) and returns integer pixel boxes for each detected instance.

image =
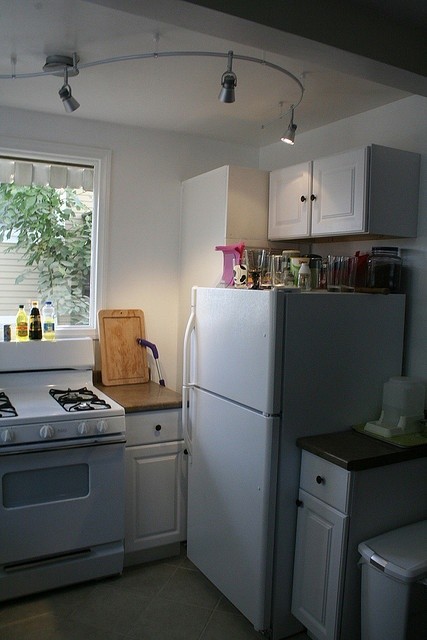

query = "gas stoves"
[0,386,125,446]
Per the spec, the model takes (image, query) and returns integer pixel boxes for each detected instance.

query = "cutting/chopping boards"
[96,309,151,386]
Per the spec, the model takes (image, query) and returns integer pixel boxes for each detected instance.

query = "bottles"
[282,250,300,288]
[29,301,42,340]
[42,301,57,341]
[16,304,29,342]
[298,262,312,292]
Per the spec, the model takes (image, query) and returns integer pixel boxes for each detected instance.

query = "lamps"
[217,55,239,106]
[55,71,82,114]
[280,107,300,148]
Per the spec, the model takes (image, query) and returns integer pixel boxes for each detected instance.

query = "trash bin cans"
[356,519,427,640]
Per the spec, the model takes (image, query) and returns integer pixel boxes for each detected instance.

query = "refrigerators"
[182,279,407,639]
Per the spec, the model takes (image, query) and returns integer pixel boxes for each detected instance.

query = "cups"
[258,255,274,289]
[311,256,327,289]
[342,256,358,292]
[246,249,265,289]
[274,255,283,286]
[232,258,246,289]
[326,255,344,292]
[289,257,310,288]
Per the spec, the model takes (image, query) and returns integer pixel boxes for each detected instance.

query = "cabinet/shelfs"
[264,143,420,244]
[125,410,187,548]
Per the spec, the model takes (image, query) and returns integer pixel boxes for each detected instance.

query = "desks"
[95,377,187,566]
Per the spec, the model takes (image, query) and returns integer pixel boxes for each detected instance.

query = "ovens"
[1,444,125,572]
